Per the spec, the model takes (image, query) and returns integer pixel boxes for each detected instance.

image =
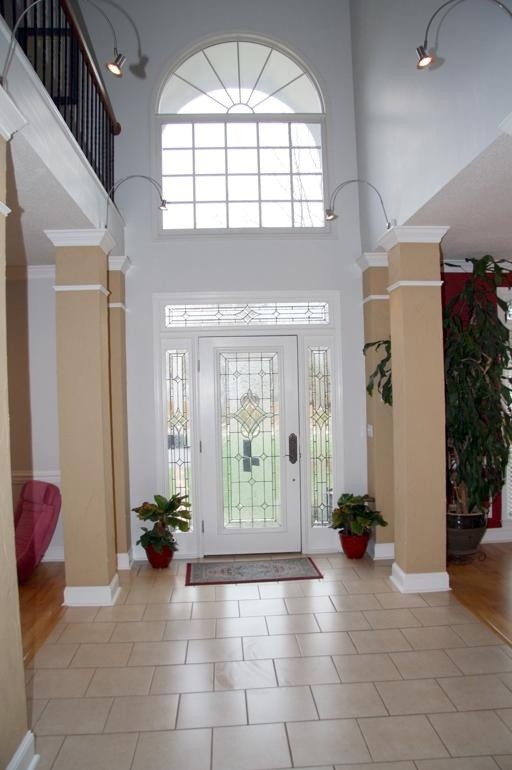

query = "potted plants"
[132,495,192,567]
[331,492,387,559]
[361,255,512,566]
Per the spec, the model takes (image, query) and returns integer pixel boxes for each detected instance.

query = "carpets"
[186,558,325,586]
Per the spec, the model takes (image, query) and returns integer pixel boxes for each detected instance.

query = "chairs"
[13,478,62,586]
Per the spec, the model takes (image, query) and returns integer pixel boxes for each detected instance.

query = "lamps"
[105,174,168,229]
[327,180,391,229]
[0,1,127,87]
[415,0,512,68]
